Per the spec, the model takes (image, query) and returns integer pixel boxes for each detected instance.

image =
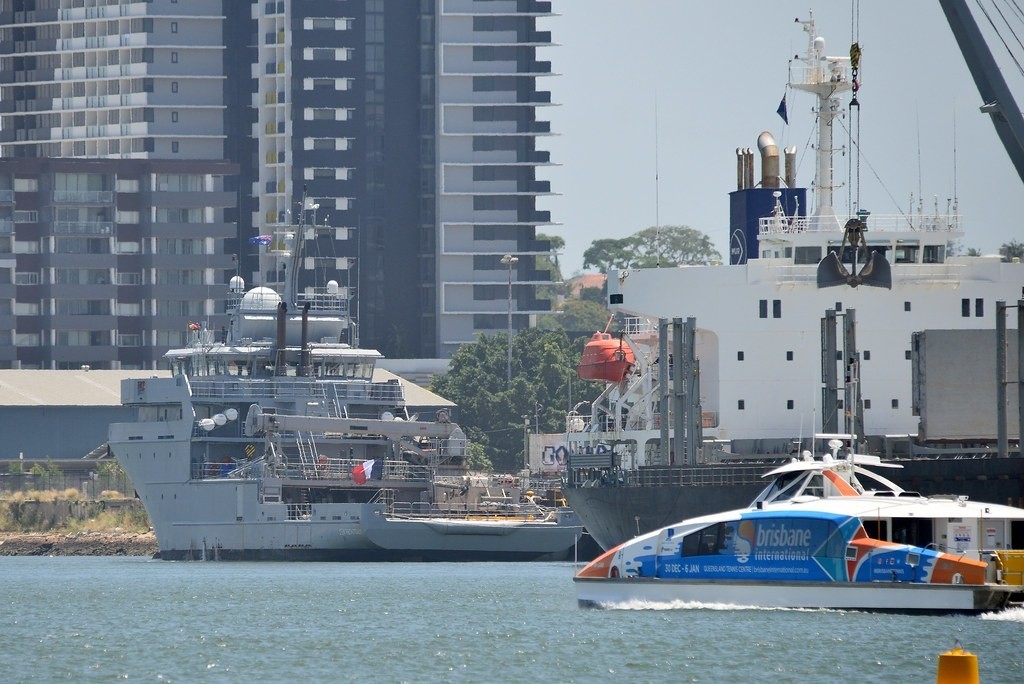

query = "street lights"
[499,254,518,389]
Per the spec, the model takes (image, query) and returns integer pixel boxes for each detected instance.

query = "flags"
[352,457,383,485]
[248,235,272,247]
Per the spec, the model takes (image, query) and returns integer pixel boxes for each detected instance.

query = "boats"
[578,333,634,384]
[588,442,1024,617]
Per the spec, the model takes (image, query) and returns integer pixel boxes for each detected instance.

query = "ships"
[106,4,1024,563]
